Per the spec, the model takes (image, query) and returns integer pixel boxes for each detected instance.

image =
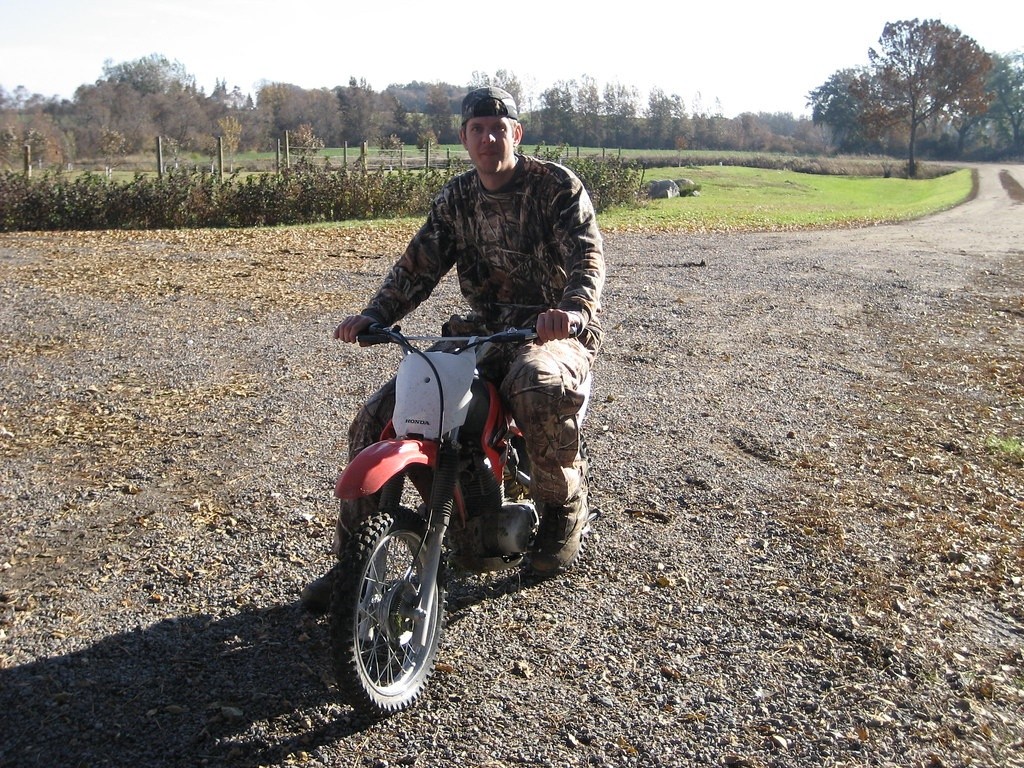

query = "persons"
[301,87,607,615]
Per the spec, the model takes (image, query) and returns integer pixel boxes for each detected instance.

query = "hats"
[462,87,517,125]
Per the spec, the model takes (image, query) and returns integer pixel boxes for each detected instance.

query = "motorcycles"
[333,323,604,719]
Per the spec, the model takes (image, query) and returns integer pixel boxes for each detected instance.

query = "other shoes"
[530,488,589,576]
[300,559,386,615]
